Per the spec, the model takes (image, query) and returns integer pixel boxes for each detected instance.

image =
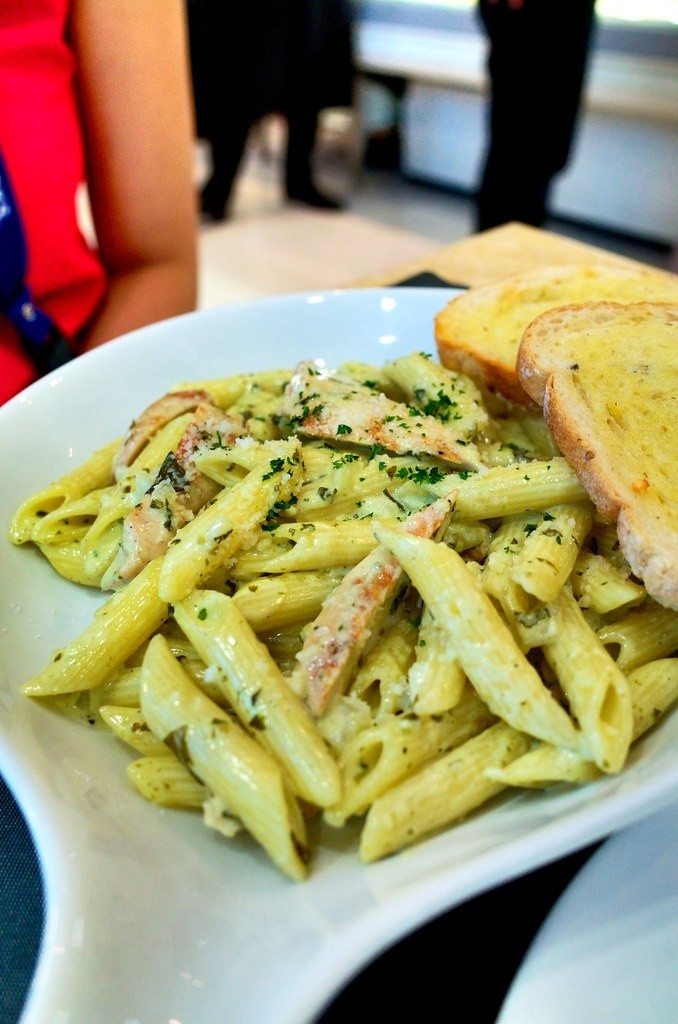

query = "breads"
[436,267,677,613]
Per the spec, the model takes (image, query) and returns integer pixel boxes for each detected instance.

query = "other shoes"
[287,182,341,209]
[199,179,233,219]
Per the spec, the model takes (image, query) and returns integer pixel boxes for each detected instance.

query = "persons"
[186,0,357,223]
[473,0,594,233]
[0,0,199,401]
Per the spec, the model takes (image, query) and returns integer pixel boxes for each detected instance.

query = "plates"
[0,286,678,1024]
